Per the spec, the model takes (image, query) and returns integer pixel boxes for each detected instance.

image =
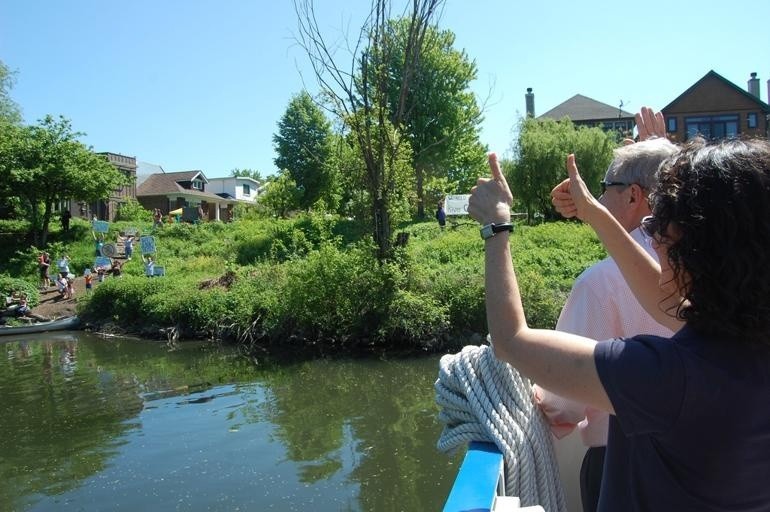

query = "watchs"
[479,222,513,239]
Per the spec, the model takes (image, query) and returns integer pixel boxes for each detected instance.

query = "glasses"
[599,178,645,195]
[639,215,681,237]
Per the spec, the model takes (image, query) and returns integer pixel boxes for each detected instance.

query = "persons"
[525,106,682,509]
[6,207,163,317]
[467,137,770,511]
[436,200,446,231]
